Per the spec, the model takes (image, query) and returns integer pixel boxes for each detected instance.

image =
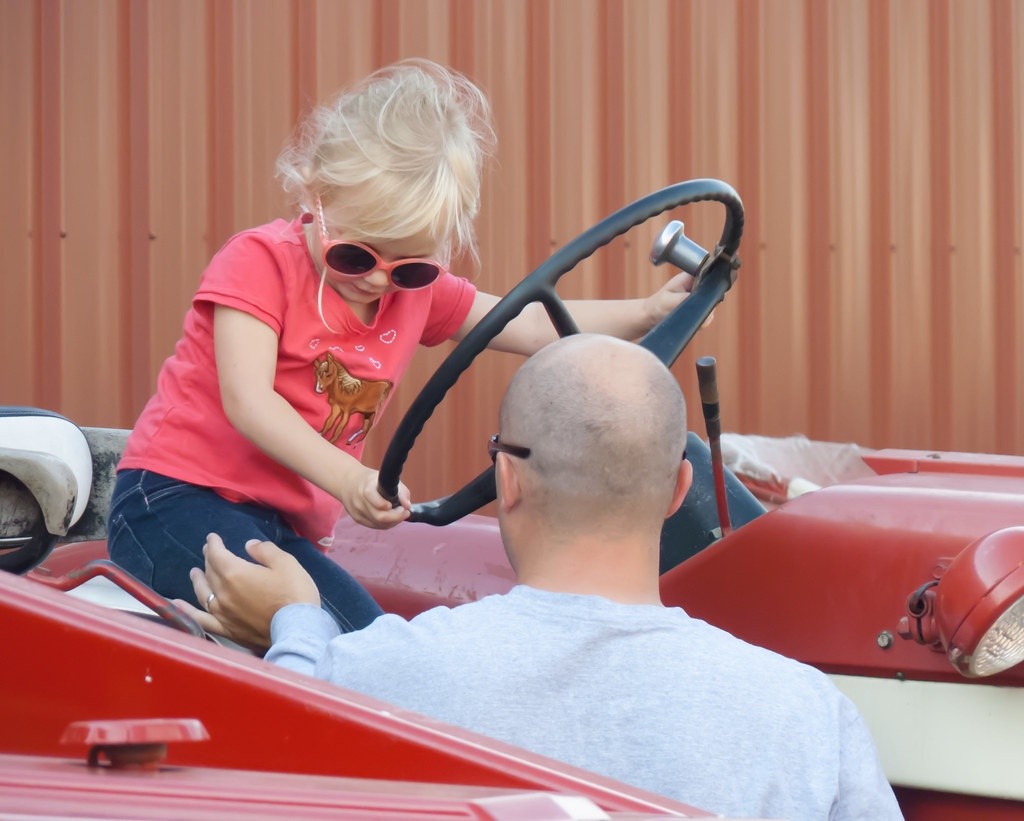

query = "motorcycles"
[2,174,1024,820]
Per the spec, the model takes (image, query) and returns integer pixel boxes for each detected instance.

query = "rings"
[205,593,215,613]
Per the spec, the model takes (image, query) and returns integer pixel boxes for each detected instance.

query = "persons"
[191,332,902,821]
[108,59,716,634]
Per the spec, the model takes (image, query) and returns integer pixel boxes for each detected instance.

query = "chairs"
[0,405,137,609]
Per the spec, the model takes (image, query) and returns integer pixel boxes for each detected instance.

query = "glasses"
[315,191,450,291]
[487,434,531,465]
